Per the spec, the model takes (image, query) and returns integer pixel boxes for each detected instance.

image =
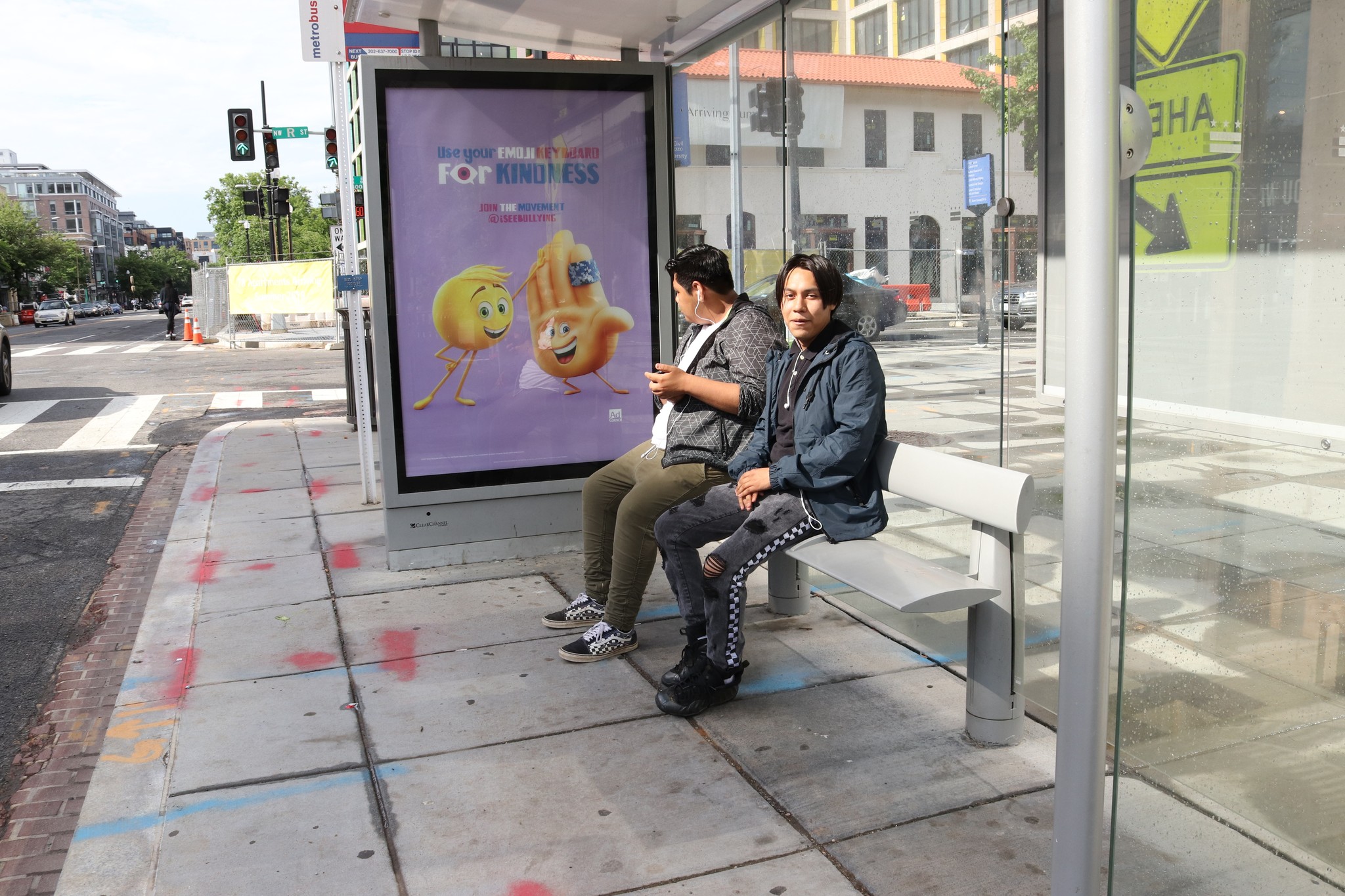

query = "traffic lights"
[228,109,255,161]
[354,192,365,219]
[243,190,263,216]
[273,188,290,216]
[262,127,279,169]
[324,127,339,169]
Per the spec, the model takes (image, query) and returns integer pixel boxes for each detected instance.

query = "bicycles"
[132,302,138,312]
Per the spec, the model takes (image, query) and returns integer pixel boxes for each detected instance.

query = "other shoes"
[165,333,176,338]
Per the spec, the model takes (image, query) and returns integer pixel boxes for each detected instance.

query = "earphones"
[784,396,790,409]
[697,290,700,301]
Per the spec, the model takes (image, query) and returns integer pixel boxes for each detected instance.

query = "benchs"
[768,438,1039,748]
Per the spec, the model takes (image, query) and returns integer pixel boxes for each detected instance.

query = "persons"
[2,304,8,312]
[161,277,181,337]
[654,254,888,717]
[542,238,789,664]
[124,298,138,309]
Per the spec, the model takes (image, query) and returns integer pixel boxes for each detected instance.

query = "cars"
[677,272,907,342]
[0,323,12,395]
[141,298,182,314]
[110,303,123,314]
[69,302,105,318]
[34,298,76,328]
[178,295,193,306]
[990,280,1037,330]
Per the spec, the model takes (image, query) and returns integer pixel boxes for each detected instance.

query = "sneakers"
[655,658,749,717]
[542,593,606,629]
[662,628,708,686]
[559,618,638,662]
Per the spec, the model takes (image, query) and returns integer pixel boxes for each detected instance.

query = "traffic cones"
[181,308,193,341]
[192,317,205,344]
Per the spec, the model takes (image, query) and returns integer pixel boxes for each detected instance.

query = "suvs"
[93,300,114,316]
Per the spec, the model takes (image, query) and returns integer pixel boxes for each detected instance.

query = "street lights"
[236,184,283,260]
[238,220,278,262]
[286,202,294,261]
[243,220,251,262]
[126,270,130,298]
[76,245,106,302]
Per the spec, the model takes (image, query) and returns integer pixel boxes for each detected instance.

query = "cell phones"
[651,370,664,384]
